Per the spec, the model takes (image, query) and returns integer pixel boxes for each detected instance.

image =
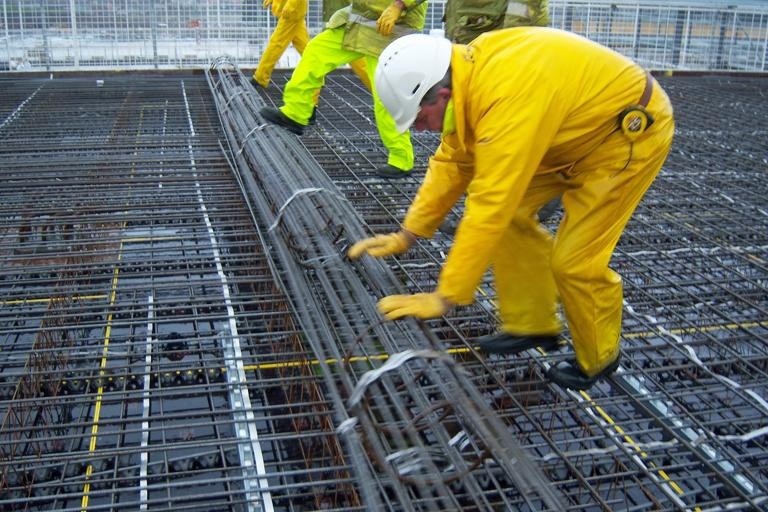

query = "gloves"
[372,1,403,35]
[372,289,450,324]
[346,229,408,262]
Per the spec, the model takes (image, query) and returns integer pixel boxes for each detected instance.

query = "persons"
[437,1,564,237]
[345,23,676,392]
[259,1,432,178]
[306,55,372,127]
[250,1,312,88]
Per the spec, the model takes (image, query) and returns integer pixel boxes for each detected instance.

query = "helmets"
[372,33,454,134]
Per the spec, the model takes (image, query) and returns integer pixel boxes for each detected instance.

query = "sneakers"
[372,164,413,180]
[547,348,626,392]
[470,322,558,356]
[258,107,305,138]
